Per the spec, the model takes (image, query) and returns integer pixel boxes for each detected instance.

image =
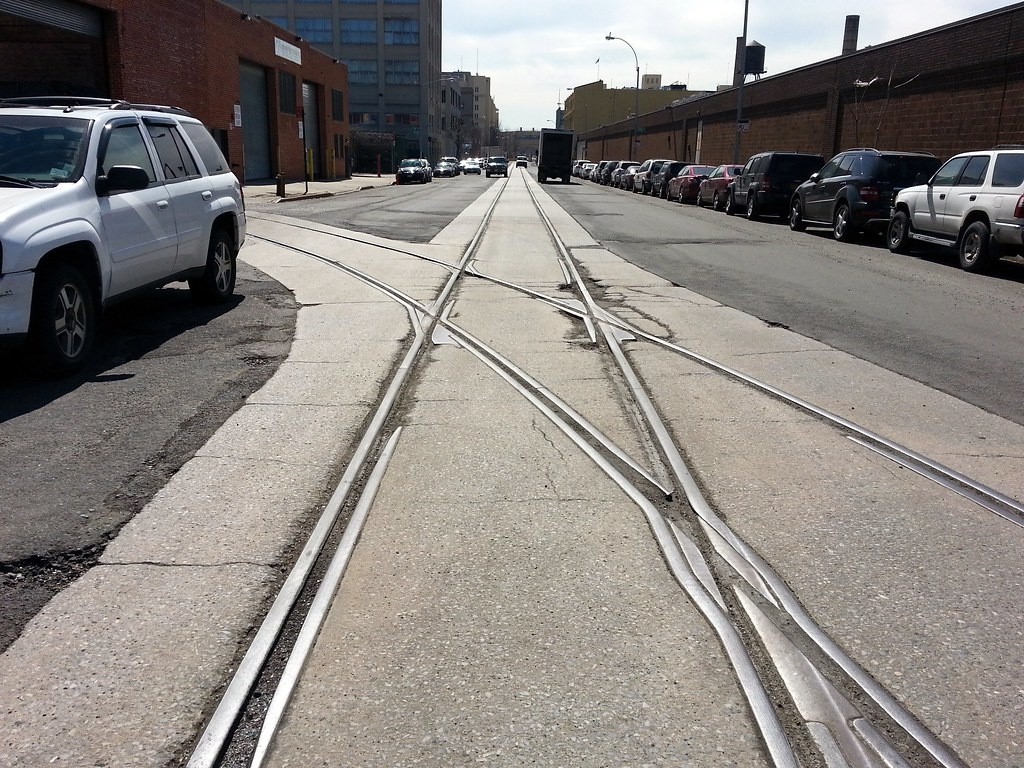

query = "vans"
[515,155,528,168]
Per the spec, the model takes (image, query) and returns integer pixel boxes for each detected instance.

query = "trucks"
[535,127,575,184]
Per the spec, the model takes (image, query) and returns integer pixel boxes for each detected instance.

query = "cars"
[572,159,598,180]
[434,156,486,178]
[396,158,428,182]
[419,158,432,182]
[696,164,745,211]
[619,165,640,190]
[486,156,510,177]
[667,165,717,203]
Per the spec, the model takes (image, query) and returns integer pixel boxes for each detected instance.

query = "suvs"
[725,150,828,220]
[651,162,697,198]
[593,160,608,183]
[887,143,1024,270]
[600,161,619,185]
[610,160,641,187]
[790,147,944,241]
[633,159,677,194]
[0,96,247,361]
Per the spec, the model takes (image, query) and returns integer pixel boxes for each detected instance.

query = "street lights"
[419,76,455,158]
[605,35,639,158]
